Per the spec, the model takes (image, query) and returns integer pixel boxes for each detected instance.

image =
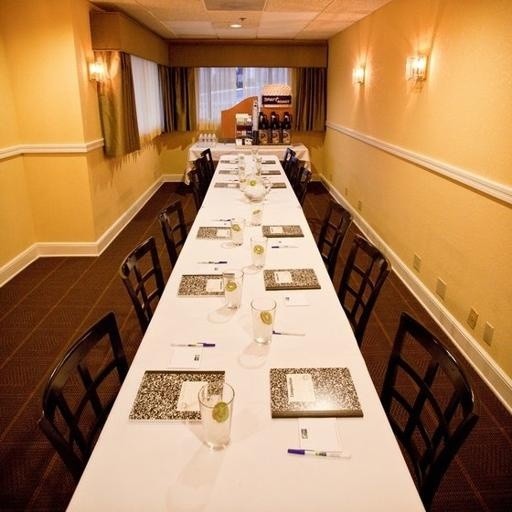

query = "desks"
[184,142,312,186]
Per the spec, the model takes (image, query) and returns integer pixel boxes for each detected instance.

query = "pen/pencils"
[171,343,215,347]
[197,261,227,264]
[288,449,351,458]
[272,245,298,247]
[273,331,306,335]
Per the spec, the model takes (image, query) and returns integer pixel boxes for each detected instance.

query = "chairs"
[295,166,312,206]
[338,234,391,348]
[380,312,478,511]
[194,157,212,186]
[118,236,166,335]
[317,199,352,279]
[187,168,207,212]
[287,156,299,187]
[283,147,296,173]
[201,148,216,176]
[160,199,188,267]
[35,312,130,484]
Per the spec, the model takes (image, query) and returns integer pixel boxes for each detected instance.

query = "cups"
[252,297,276,344]
[198,378,233,450]
[222,267,245,310]
[251,231,268,266]
[229,145,270,248]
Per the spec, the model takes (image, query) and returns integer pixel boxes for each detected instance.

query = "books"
[213,158,286,189]
[263,269,321,290]
[269,367,364,419]
[178,274,235,297]
[128,369,225,422]
[262,225,304,238]
[196,226,232,240]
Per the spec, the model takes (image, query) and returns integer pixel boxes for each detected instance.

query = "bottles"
[199,133,217,148]
[258,112,290,144]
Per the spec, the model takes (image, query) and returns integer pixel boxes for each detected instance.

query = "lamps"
[351,63,365,84]
[89,60,106,81]
[405,55,427,80]
